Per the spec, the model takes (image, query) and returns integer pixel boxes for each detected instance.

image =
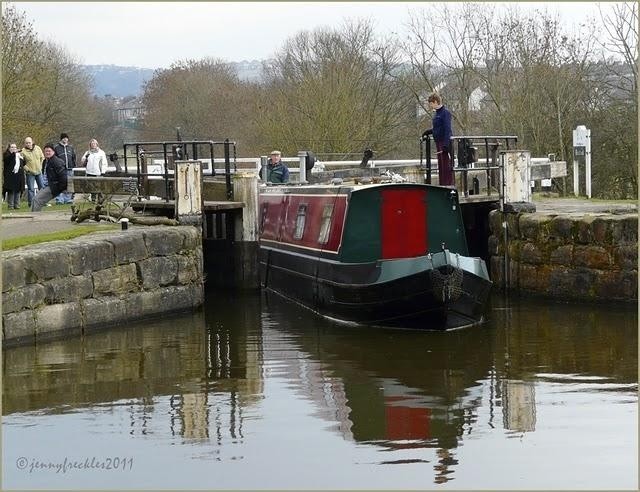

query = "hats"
[43,144,55,152]
[270,150,281,155]
[60,132,68,140]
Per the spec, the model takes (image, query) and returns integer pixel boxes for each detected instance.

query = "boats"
[259,182,495,334]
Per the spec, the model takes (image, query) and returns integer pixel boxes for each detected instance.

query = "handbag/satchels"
[83,154,90,166]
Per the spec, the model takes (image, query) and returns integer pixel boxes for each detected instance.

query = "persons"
[54,131,77,205]
[30,144,67,212]
[20,135,52,208]
[3,143,25,210]
[80,138,109,202]
[421,92,454,185]
[258,149,290,186]
